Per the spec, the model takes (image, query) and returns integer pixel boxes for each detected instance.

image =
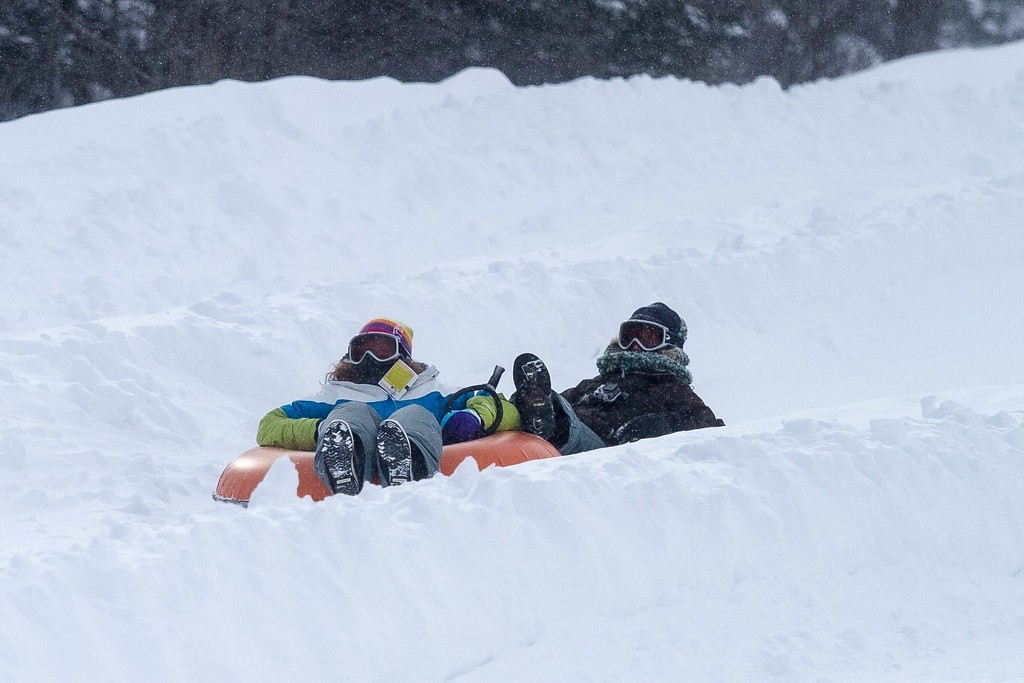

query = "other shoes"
[512,352,557,439]
[376,419,414,486]
[320,419,365,496]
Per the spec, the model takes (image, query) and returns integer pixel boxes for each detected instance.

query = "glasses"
[348,331,400,364]
[617,319,669,351]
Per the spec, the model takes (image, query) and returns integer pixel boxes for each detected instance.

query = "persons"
[257,318,521,497]
[510,302,727,458]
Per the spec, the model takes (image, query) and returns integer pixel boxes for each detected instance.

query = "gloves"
[440,408,482,443]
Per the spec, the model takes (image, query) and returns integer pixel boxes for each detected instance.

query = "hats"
[629,302,688,341]
[359,318,414,357]
